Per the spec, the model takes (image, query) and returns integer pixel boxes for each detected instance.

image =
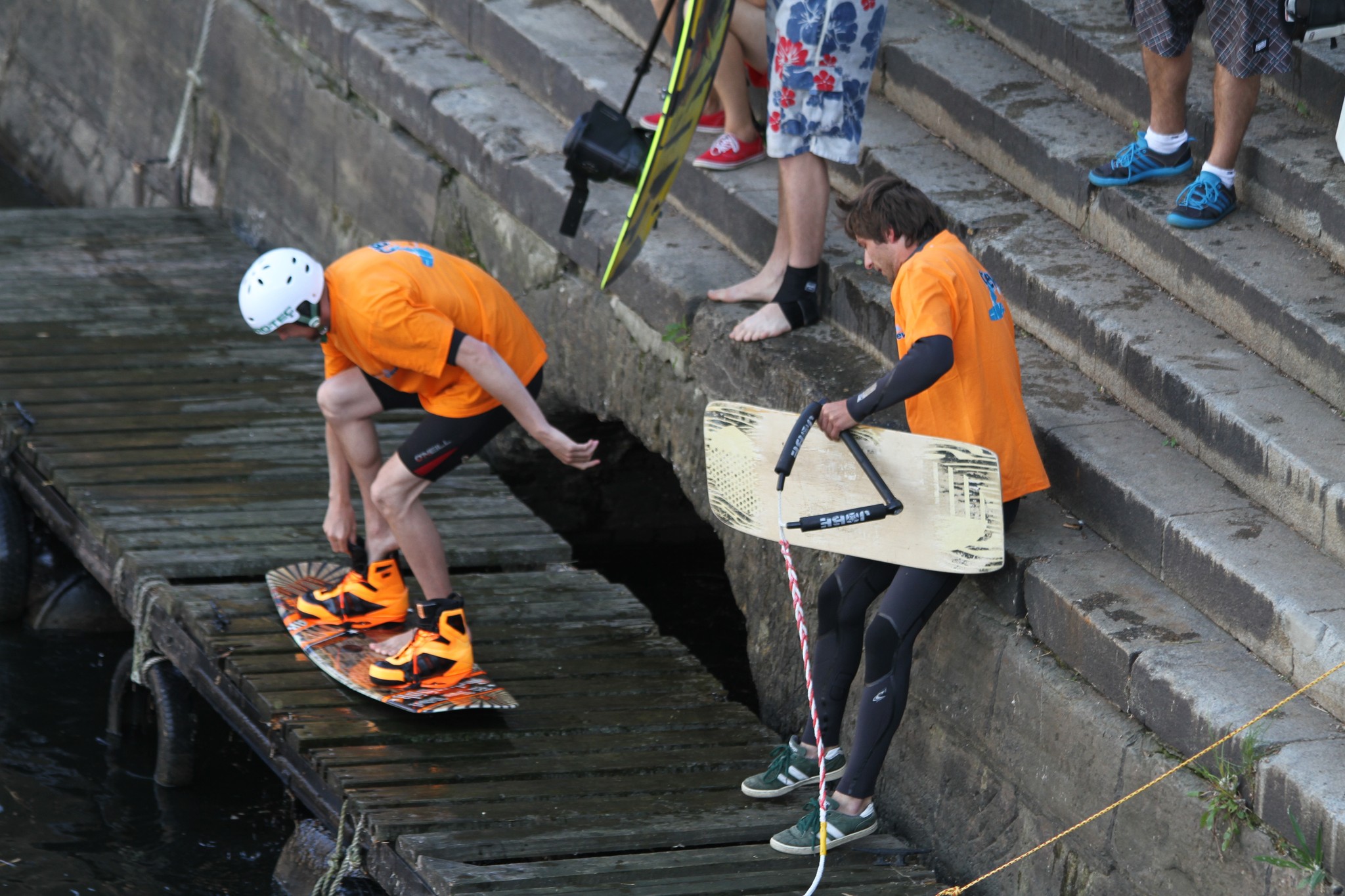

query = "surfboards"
[599,0,735,291]
[265,560,520,714]
[696,399,1005,574]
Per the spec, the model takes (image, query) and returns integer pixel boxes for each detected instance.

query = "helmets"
[238,248,323,335]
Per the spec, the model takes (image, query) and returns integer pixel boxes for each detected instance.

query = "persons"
[1088,0,1292,230]
[640,0,778,171]
[707,0,887,342]
[239,239,600,686]
[739,176,1049,855]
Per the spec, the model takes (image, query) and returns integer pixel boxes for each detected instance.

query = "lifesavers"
[107,648,195,788]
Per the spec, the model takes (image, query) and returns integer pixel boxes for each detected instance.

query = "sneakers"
[367,596,473,685]
[742,734,845,797]
[692,133,766,170]
[1167,170,1237,230]
[296,559,410,628]
[769,801,877,856]
[638,110,725,134]
[1088,132,1198,187]
